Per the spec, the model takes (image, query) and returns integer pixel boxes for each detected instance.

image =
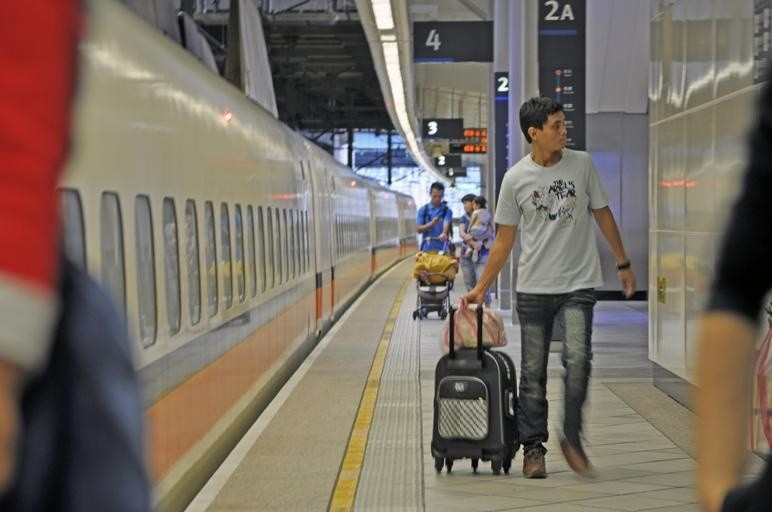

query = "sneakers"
[465,248,472,259]
[472,250,479,262]
[560,439,588,475]
[523,448,545,478]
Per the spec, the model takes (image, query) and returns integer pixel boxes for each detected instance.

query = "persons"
[463,98,636,479]
[416,181,453,252]
[458,194,493,307]
[464,196,491,262]
[688,65,772,512]
[1,0,89,495]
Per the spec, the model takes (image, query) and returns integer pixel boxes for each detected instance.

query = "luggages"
[431,304,520,475]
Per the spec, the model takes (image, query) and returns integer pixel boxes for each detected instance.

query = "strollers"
[412,237,456,320]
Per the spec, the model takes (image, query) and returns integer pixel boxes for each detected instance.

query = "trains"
[57,0,419,510]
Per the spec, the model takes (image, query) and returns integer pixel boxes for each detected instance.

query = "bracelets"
[617,260,631,270]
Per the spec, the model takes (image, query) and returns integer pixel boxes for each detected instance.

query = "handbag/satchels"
[444,308,508,348]
[412,251,458,284]
